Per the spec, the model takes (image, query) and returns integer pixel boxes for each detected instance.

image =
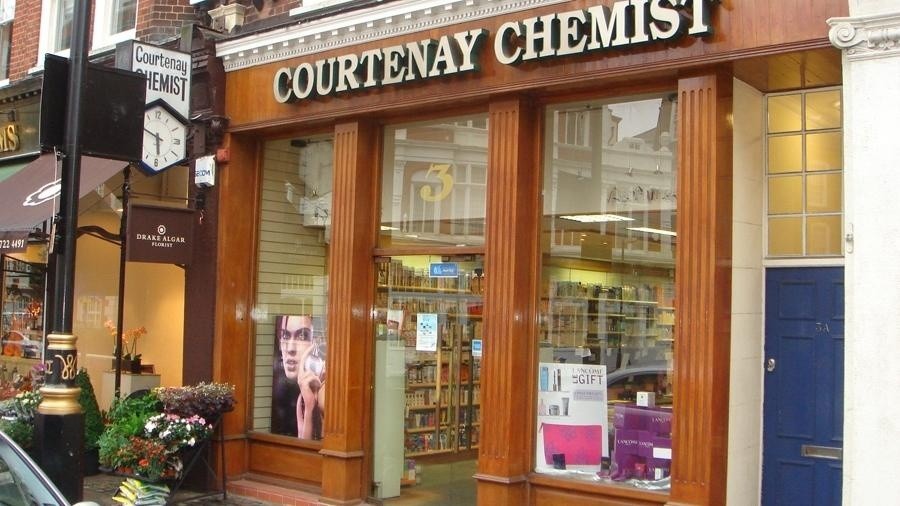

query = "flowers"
[96,380,236,489]
[105,320,147,360]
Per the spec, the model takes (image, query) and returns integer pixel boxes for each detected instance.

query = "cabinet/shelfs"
[372,285,482,462]
[541,297,675,372]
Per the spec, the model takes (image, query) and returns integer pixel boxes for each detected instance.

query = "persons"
[273,313,326,441]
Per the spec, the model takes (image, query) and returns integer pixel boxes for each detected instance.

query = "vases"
[112,359,140,373]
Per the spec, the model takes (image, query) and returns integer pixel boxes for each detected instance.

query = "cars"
[0,432,98,506]
[1,330,42,356]
[603,359,674,467]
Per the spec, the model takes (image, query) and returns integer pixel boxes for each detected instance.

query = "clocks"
[132,98,189,176]
